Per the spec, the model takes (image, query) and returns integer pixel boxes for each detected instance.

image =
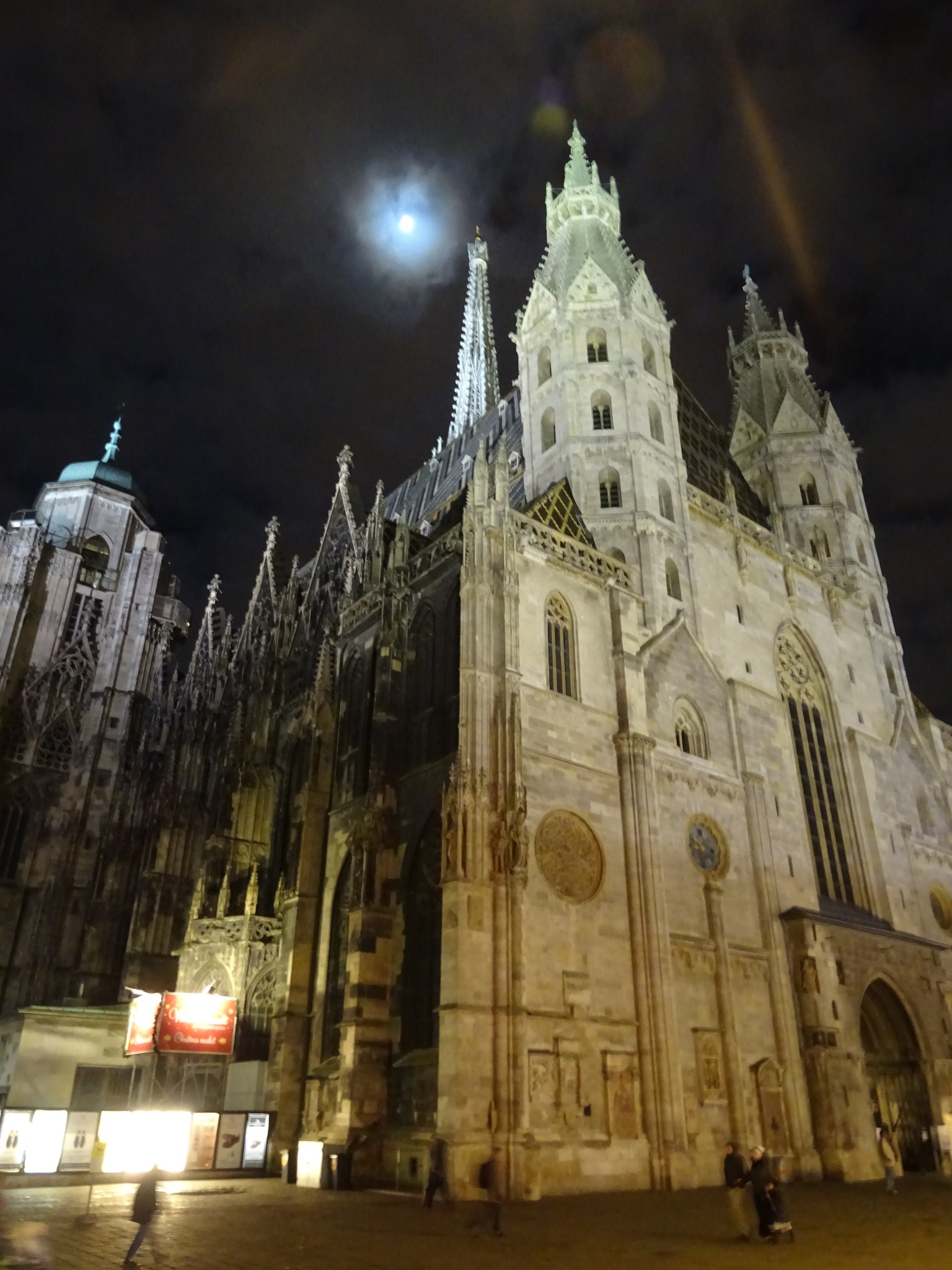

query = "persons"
[736,1145,795,1245]
[878,1125,900,1195]
[723,1139,749,1242]
[464,1146,508,1239]
[122,1168,163,1269]
[423,1137,453,1209]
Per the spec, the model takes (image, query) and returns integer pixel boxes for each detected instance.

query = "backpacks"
[477,1161,495,1190]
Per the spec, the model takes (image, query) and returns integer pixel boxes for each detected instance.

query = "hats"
[726,1142,740,1153]
[754,1145,766,1154]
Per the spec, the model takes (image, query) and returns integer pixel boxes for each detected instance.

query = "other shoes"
[123,1259,137,1267]
[730,1235,749,1244]
